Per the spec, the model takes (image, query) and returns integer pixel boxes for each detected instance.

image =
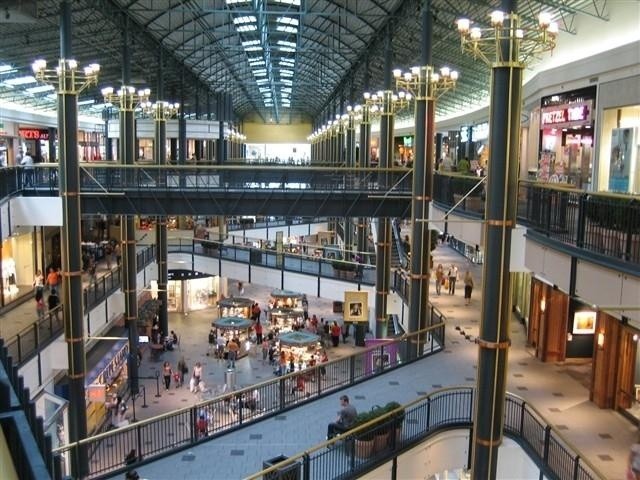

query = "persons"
[463,271,475,306]
[126,470,149,480]
[341,322,348,344]
[325,394,357,442]
[435,264,444,295]
[15,150,24,165]
[156,294,341,437]
[398,225,411,242]
[455,157,469,173]
[475,164,484,177]
[20,151,34,186]
[115,394,129,423]
[351,254,365,277]
[447,263,459,295]
[110,392,118,427]
[125,448,137,465]
[32,241,122,329]
[238,282,244,295]
[438,158,445,171]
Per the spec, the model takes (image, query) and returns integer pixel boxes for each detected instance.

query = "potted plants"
[354,401,405,457]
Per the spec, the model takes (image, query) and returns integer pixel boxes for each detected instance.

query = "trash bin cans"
[263,454,302,480]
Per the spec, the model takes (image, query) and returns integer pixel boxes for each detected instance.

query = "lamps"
[305,9,558,146]
[141,99,182,122]
[100,86,152,114]
[225,127,247,145]
[29,58,103,95]
